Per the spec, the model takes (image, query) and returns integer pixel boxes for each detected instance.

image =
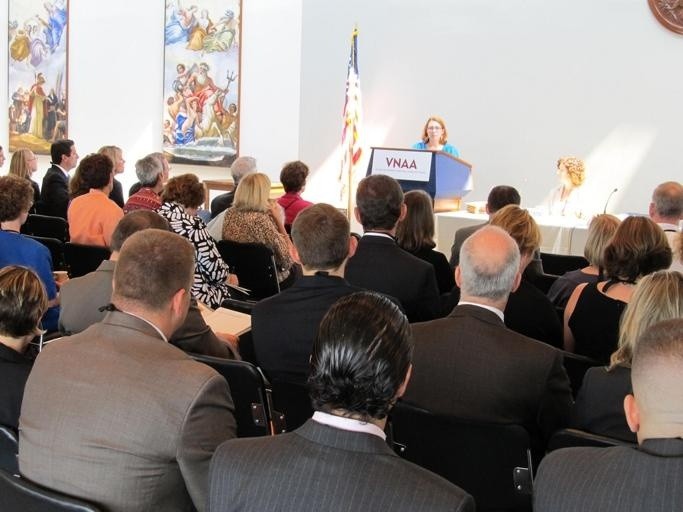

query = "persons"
[204,289,476,511]
[404,113,459,159]
[0,138,682,440]
[8,0,65,144]
[520,318,682,512]
[163,1,237,146]
[13,226,238,511]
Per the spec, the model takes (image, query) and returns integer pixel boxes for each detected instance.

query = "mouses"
[604,187,618,215]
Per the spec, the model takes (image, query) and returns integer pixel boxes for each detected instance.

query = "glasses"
[427,126,444,131]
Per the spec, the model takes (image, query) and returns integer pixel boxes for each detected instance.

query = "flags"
[334,27,362,202]
